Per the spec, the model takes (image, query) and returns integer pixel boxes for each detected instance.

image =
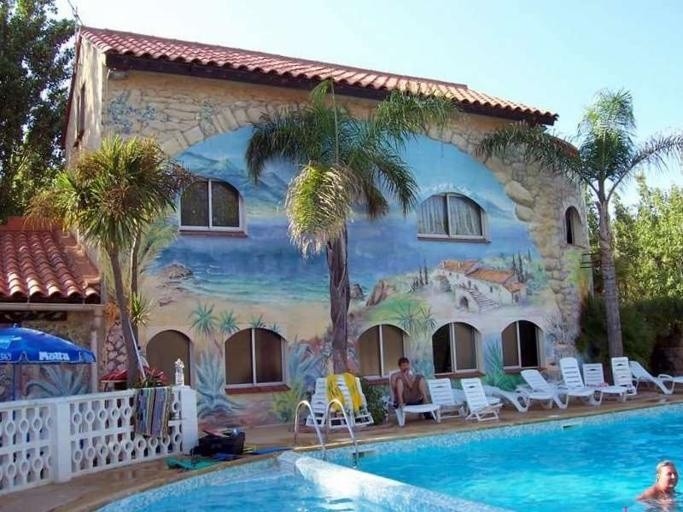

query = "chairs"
[388,367,441,426]
[425,377,467,423]
[629,358,682,396]
[482,382,531,417]
[582,363,627,404]
[611,358,638,401]
[556,356,601,407]
[515,366,576,411]
[459,377,505,423]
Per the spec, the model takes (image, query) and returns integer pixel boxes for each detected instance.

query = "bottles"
[174,358,184,386]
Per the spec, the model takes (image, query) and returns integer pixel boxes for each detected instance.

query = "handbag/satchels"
[189,432,246,457]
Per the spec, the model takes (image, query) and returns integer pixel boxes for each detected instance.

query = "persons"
[637,460,679,501]
[391,357,429,407]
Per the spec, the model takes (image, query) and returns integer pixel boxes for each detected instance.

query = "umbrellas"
[0,323,96,400]
